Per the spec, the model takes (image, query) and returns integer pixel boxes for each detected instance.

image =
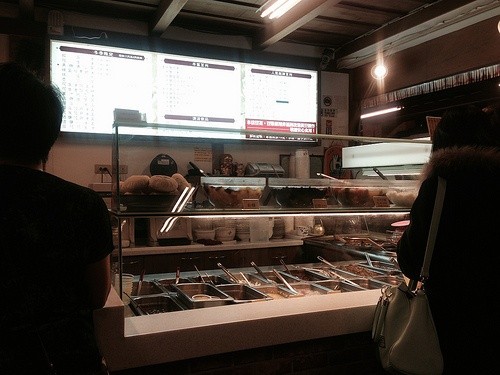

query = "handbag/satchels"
[371,282,442,375]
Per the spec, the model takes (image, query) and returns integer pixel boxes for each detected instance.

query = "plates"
[286,233,313,239]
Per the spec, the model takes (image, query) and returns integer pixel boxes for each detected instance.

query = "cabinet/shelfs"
[111,121,433,318]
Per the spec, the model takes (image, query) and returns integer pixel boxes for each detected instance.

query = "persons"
[0,62,114,375]
[396,109,500,375]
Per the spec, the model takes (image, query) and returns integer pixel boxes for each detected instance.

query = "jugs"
[249,216,269,243]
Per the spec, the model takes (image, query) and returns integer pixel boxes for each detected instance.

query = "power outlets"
[95,164,128,175]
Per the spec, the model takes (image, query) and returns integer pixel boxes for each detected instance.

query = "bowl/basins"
[193,218,314,242]
[386,180,423,207]
[118,192,188,212]
[200,176,266,208]
[329,179,390,207]
[268,178,330,207]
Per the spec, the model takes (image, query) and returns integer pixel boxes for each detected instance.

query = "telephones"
[246,160,286,176]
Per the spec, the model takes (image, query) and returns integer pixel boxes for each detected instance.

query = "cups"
[115,273,134,305]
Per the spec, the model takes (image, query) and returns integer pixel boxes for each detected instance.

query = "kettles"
[307,217,325,234]
